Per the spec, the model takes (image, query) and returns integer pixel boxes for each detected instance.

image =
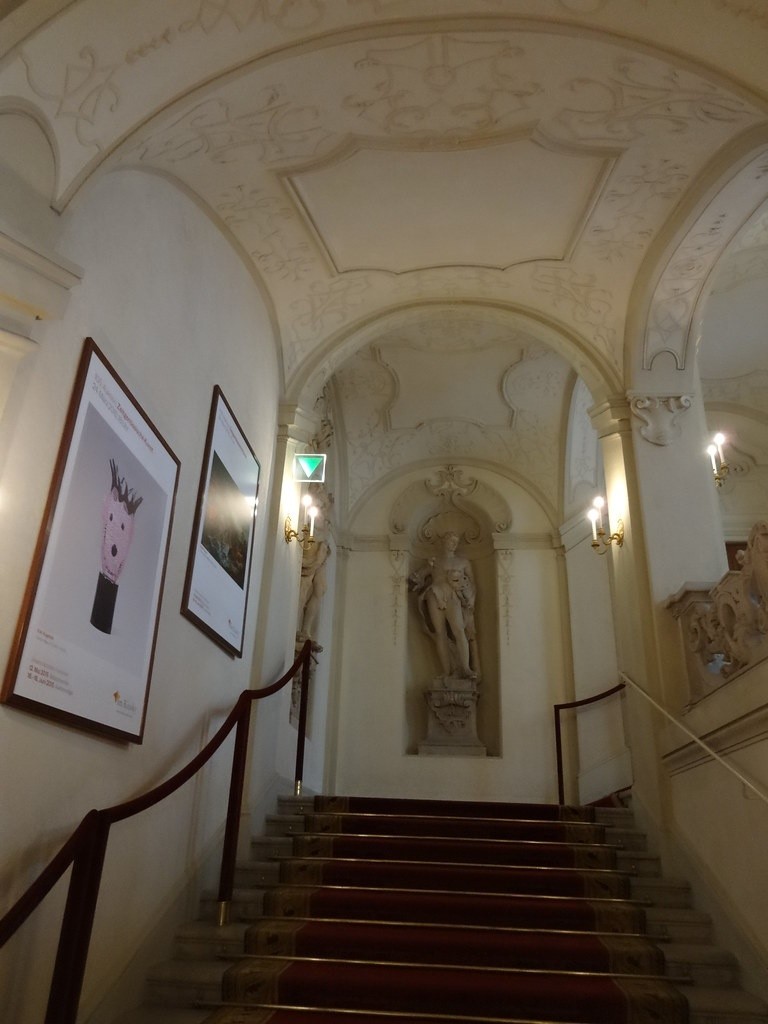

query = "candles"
[706,445,717,474]
[714,433,725,463]
[593,496,604,529]
[307,507,318,536]
[588,509,599,540]
[302,494,312,524]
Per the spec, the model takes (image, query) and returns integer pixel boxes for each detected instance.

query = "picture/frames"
[0,336,182,745]
[168,384,262,659]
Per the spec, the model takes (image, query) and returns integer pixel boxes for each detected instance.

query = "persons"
[296,474,334,641]
[412,531,479,679]
